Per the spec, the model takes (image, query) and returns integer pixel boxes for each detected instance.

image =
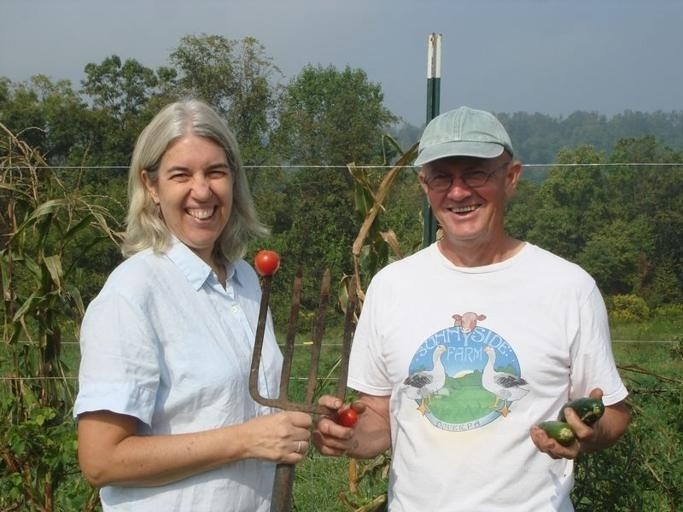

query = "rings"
[296,440,302,453]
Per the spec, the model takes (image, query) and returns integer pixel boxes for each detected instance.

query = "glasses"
[423,161,510,192]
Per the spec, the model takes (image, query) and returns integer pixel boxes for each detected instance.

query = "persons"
[309,107,631,512]
[73,98,311,511]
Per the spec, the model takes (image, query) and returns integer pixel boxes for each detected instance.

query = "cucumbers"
[537,421,575,447]
[557,398,605,426]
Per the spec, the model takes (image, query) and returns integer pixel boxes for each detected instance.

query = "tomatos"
[339,406,358,427]
[254,250,280,277]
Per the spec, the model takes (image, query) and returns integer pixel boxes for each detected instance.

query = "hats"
[414,105,514,167]
